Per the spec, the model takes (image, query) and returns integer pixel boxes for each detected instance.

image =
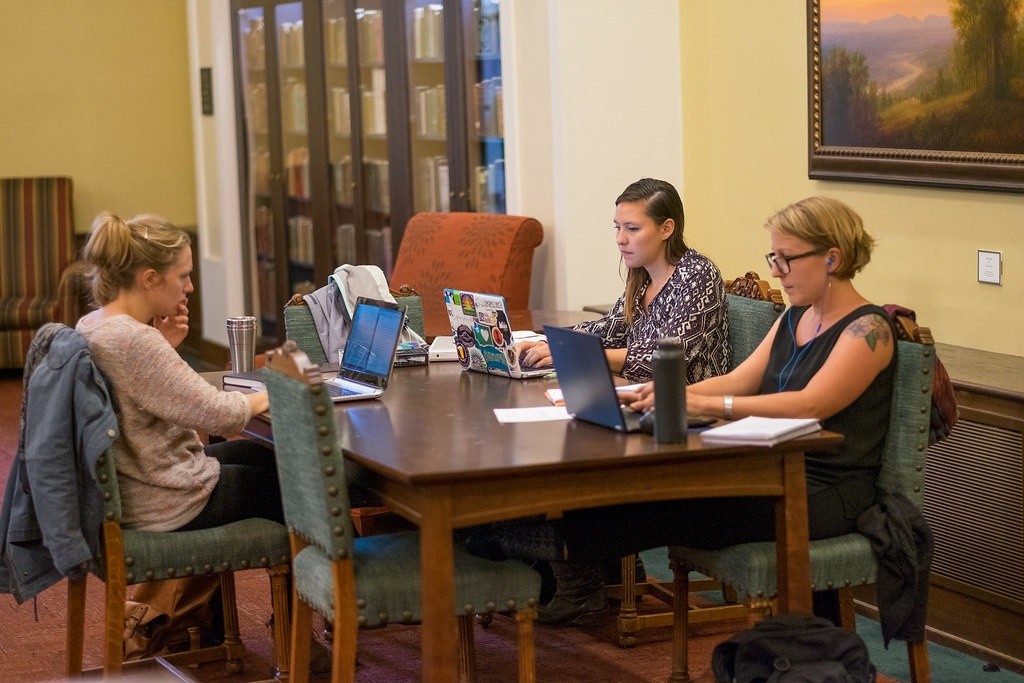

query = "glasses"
[765,248,826,274]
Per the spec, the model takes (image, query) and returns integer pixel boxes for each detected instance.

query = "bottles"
[652,338,688,444]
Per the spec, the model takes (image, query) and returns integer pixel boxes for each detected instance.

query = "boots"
[536,558,612,629]
[464,520,561,561]
[266,613,334,673]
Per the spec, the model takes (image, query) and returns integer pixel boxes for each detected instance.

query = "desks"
[189,369,843,683]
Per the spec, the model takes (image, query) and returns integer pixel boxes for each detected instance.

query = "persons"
[73,209,363,680]
[533,194,899,629]
[515,176,733,603]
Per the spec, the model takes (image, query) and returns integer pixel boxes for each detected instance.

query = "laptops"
[443,287,556,379]
[326,296,408,401]
[542,323,719,433]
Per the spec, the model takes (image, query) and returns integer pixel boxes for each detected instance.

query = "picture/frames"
[806,0,1024,192]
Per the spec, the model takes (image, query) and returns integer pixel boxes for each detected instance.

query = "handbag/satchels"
[713,614,877,683]
[123,573,224,663]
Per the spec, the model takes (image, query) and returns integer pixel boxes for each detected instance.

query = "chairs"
[37,324,540,683]
[263,215,931,683]
[0,177,82,368]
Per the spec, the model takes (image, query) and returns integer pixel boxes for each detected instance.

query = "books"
[256,205,275,259]
[415,154,506,213]
[335,224,355,266]
[413,2,503,139]
[243,8,387,135]
[254,146,389,215]
[362,225,392,281]
[699,416,822,448]
[287,218,314,265]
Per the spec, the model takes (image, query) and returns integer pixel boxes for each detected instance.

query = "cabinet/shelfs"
[231,0,501,355]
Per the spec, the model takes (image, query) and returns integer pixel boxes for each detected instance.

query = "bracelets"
[723,395,734,420]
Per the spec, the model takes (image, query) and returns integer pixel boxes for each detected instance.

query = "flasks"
[225,315,257,375]
